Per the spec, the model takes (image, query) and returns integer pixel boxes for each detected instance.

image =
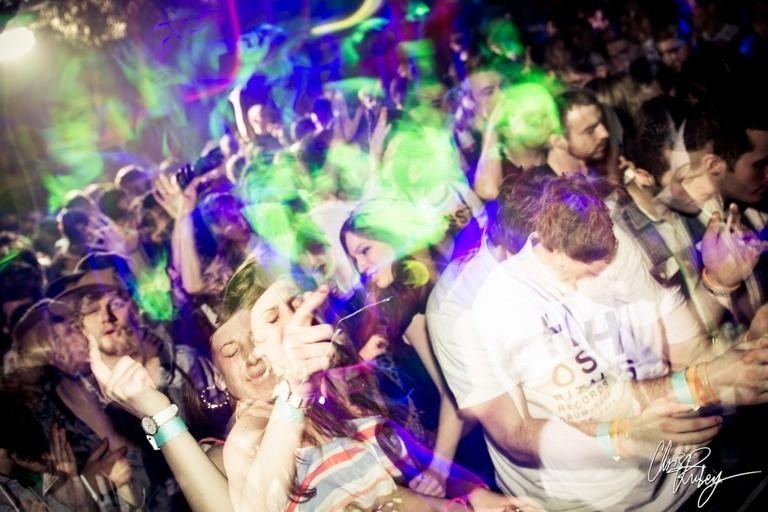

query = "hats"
[39,248,139,319]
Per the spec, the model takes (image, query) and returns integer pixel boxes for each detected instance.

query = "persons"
[0,1,768,512]
